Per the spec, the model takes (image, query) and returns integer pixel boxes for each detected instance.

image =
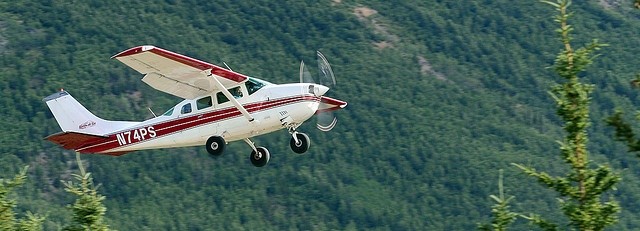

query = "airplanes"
[42,45,347,167]
